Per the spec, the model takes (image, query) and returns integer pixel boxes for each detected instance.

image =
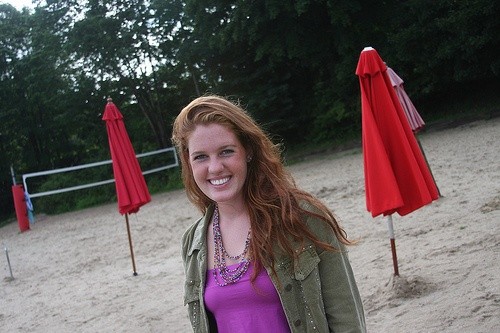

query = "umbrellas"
[102,96,152,276]
[383,62,425,133]
[356,46,444,276]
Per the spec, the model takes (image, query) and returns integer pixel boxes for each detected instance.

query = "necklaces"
[213,204,252,286]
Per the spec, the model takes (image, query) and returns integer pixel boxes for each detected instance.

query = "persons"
[172,96,368,333]
